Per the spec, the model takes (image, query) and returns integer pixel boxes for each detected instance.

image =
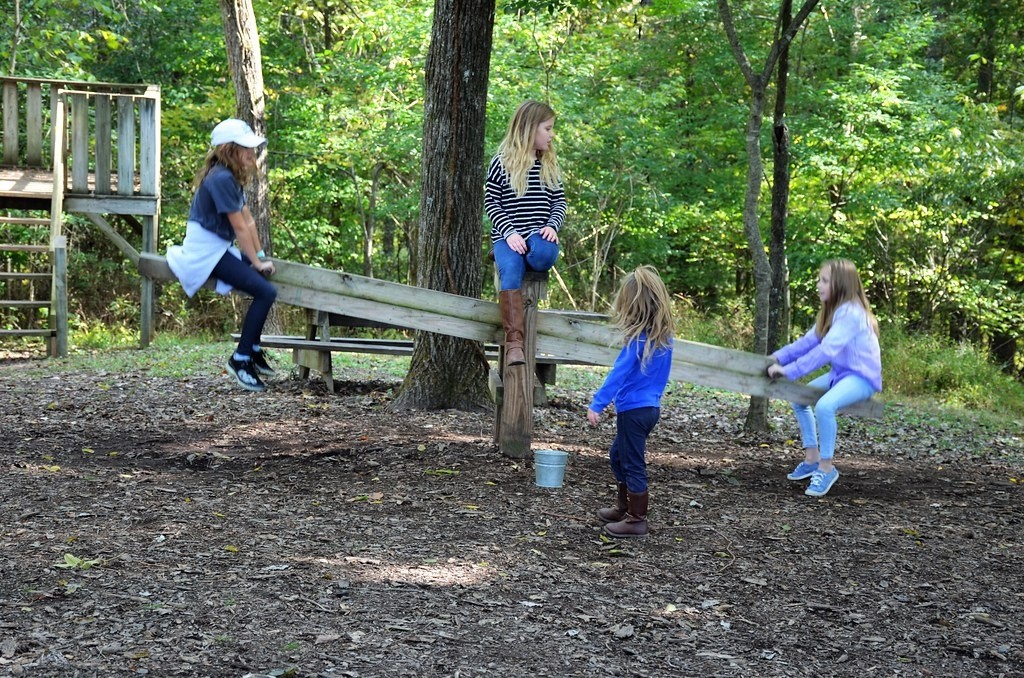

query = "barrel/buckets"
[531,449,569,486]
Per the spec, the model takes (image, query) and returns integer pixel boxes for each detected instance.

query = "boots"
[499,289,528,367]
[597,481,649,538]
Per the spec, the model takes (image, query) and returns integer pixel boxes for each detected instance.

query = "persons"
[586,264,674,537]
[767,257,882,497]
[484,101,566,366]
[166,118,277,391]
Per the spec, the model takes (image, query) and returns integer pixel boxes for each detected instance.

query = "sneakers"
[227,349,276,391]
[787,459,840,497]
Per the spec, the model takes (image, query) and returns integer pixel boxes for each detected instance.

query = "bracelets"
[251,256,260,264]
[257,250,265,258]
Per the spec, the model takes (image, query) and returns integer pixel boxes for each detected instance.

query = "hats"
[210,119,267,149]
